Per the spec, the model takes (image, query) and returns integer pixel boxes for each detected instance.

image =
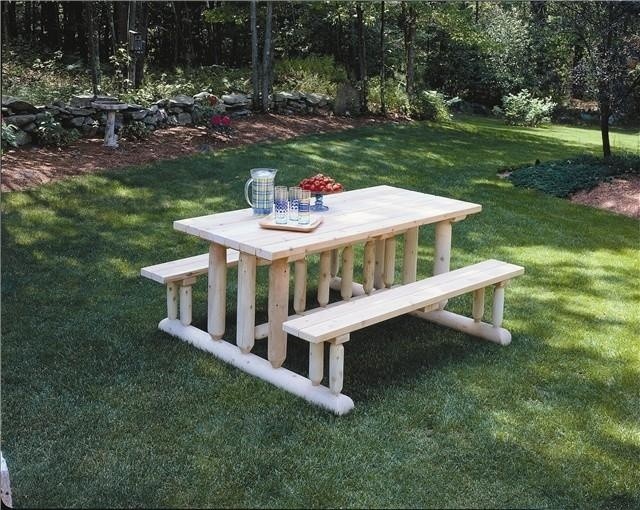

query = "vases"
[309,191,328,211]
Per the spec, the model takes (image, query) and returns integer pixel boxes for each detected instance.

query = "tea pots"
[244,168,277,214]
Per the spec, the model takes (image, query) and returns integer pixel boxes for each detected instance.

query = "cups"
[274,184,312,225]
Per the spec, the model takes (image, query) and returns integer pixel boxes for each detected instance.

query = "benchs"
[281,257,523,394]
[141,248,239,328]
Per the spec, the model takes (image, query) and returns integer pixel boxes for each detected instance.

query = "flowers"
[297,174,343,192]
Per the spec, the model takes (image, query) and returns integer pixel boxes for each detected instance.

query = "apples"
[298,173,342,192]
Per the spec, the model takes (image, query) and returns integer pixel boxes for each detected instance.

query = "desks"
[159,184,512,412]
